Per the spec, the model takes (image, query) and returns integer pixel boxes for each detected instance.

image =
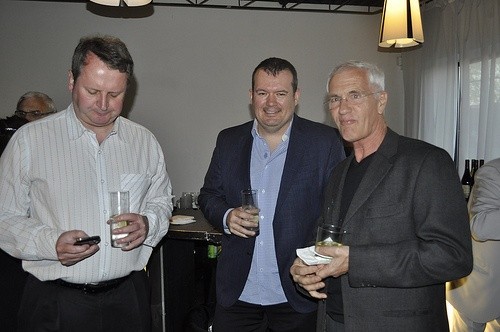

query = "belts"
[56,271,136,295]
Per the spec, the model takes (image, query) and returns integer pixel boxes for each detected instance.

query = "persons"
[290,59,473,332]
[446,157,500,332]
[197,57,347,332]
[0,32,173,332]
[15,92,57,122]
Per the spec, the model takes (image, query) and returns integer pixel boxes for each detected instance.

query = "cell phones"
[74,235,100,246]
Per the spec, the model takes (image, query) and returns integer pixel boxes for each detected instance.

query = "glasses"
[15,110,55,119]
[325,90,381,108]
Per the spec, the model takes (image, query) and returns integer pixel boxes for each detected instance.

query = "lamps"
[377,0,424,48]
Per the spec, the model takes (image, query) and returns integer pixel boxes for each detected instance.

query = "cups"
[241,189,260,237]
[180,197,191,210]
[110,191,130,248]
[315,224,347,258]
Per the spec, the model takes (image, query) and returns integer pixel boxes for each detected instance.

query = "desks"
[158,207,223,332]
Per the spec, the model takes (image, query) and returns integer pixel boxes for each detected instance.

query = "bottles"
[480,159,484,168]
[460,160,478,204]
